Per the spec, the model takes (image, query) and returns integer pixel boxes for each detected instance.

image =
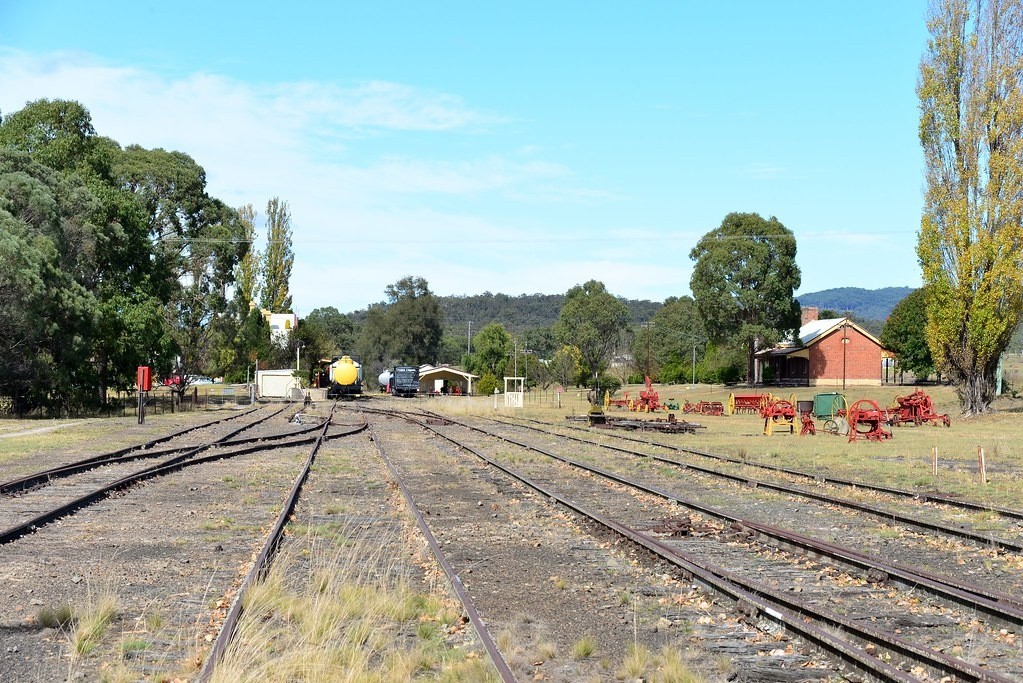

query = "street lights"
[297,341,305,369]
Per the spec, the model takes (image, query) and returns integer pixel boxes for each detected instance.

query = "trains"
[327,356,362,399]
[379,367,419,397]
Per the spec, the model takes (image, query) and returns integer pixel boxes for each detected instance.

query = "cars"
[164,375,191,388]
